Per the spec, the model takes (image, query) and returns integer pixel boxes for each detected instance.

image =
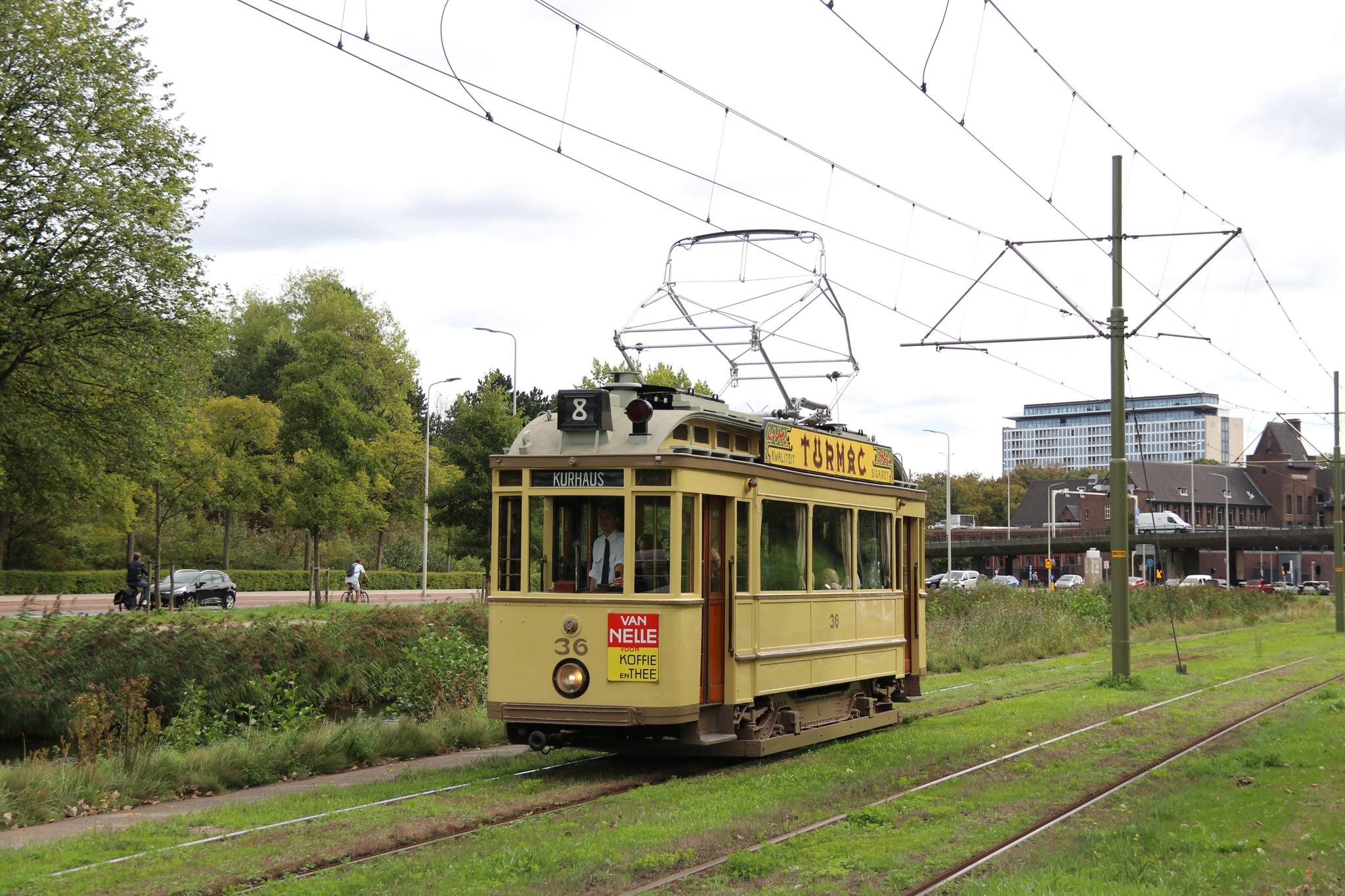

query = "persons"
[588,505,670,594]
[125,552,155,610]
[979,570,985,576]
[1032,571,1039,588]
[345,559,369,603]
[681,506,887,591]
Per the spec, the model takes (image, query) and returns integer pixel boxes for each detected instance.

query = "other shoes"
[346,598,349,602]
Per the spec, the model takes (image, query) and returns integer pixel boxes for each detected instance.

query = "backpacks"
[345,563,360,577]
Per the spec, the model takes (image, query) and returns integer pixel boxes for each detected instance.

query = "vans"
[1179,575,1212,588]
[1137,511,1193,535]
[939,570,979,591]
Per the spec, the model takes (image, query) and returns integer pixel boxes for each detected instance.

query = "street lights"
[472,327,516,417]
[1210,473,1229,591]
[1311,561,1316,581]
[1048,482,1066,589]
[938,452,955,542]
[1181,441,1203,533]
[1002,463,1010,540]
[922,429,952,590]
[423,377,462,598]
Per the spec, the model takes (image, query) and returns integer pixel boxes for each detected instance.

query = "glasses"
[820,577,830,580]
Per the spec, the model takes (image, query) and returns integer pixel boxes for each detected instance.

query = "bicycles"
[119,576,155,612]
[341,580,369,604]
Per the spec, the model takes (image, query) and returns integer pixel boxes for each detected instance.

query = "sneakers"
[142,600,152,605]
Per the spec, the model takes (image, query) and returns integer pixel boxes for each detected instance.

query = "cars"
[1160,578,1182,588]
[1128,576,1147,589]
[1054,574,1084,592]
[1231,579,1330,595]
[991,575,1019,591]
[924,573,946,590]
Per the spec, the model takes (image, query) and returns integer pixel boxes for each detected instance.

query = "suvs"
[1204,578,1235,590]
[141,569,237,611]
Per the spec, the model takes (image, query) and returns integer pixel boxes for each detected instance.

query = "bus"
[486,229,927,759]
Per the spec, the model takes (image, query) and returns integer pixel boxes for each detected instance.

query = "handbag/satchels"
[114,589,134,609]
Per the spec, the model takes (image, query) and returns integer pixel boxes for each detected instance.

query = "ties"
[602,539,610,585]
